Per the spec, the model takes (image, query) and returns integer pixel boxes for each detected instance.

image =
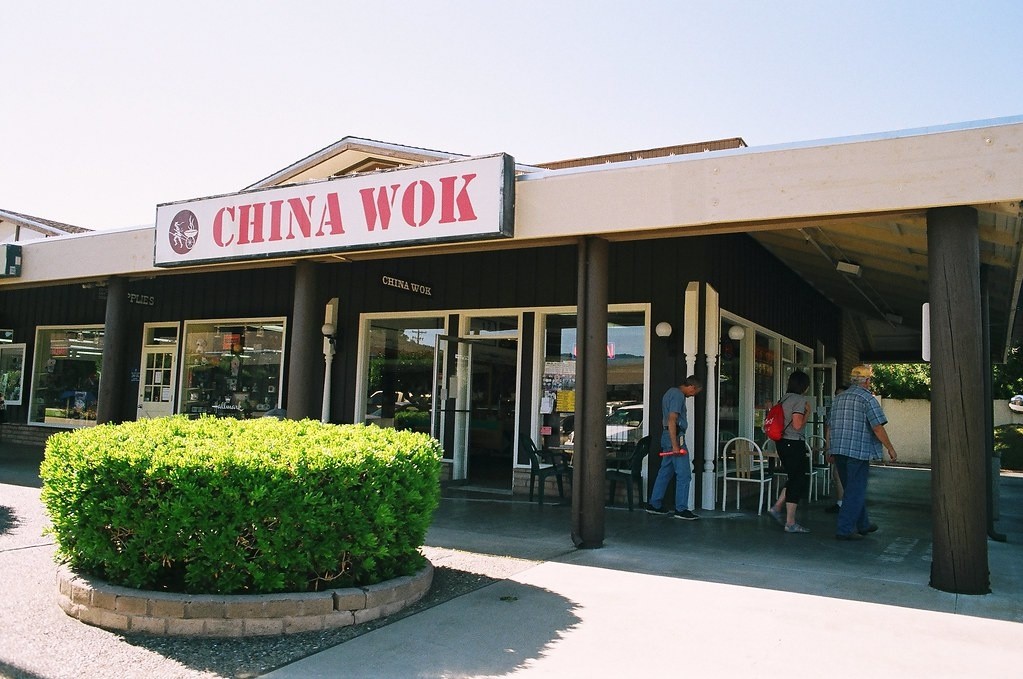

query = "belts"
[664,426,668,430]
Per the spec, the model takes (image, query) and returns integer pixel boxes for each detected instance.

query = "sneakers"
[646,505,669,515]
[673,509,699,520]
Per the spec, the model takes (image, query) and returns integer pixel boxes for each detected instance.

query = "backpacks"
[764,393,800,440]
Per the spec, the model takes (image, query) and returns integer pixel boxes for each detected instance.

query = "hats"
[851,366,875,378]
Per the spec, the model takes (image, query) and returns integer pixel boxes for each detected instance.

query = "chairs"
[516,429,830,519]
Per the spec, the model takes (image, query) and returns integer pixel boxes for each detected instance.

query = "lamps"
[729,325,745,349]
[321,323,338,346]
[655,322,672,345]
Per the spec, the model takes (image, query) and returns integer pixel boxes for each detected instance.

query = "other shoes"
[825,504,841,514]
[837,533,863,540]
[859,524,878,535]
[770,506,785,526]
[784,524,810,533]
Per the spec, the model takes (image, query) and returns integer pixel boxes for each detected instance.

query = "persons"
[646,375,703,520]
[825,367,897,540]
[768,371,812,532]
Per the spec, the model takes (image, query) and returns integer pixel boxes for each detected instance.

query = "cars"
[541,413,574,449]
[367,398,378,413]
[605,404,620,418]
[261,408,287,422]
[365,402,411,419]
[369,390,409,408]
[607,401,624,408]
[562,404,644,457]
